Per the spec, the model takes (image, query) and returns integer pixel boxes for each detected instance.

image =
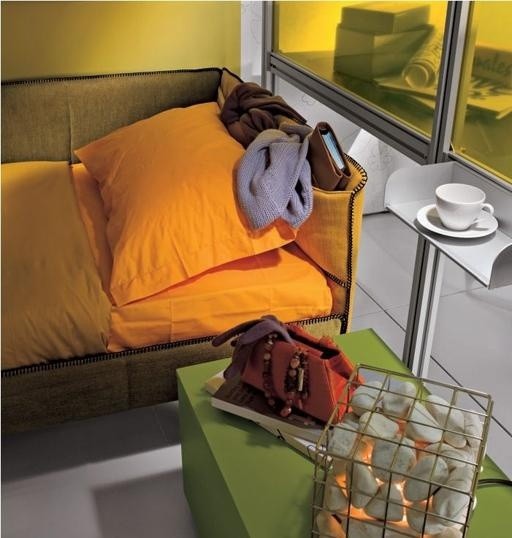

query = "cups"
[436,182,494,231]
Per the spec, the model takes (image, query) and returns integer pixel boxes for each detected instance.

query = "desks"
[176,328,512,538]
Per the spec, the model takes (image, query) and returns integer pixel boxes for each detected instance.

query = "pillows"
[75,101,300,307]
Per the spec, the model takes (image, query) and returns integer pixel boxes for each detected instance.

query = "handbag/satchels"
[214,314,363,423]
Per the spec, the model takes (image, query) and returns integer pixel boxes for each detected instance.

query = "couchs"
[0,68,367,442]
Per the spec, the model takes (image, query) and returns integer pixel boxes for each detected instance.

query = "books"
[204,362,334,470]
[209,373,334,447]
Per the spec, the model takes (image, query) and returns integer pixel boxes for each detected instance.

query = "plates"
[417,204,499,239]
